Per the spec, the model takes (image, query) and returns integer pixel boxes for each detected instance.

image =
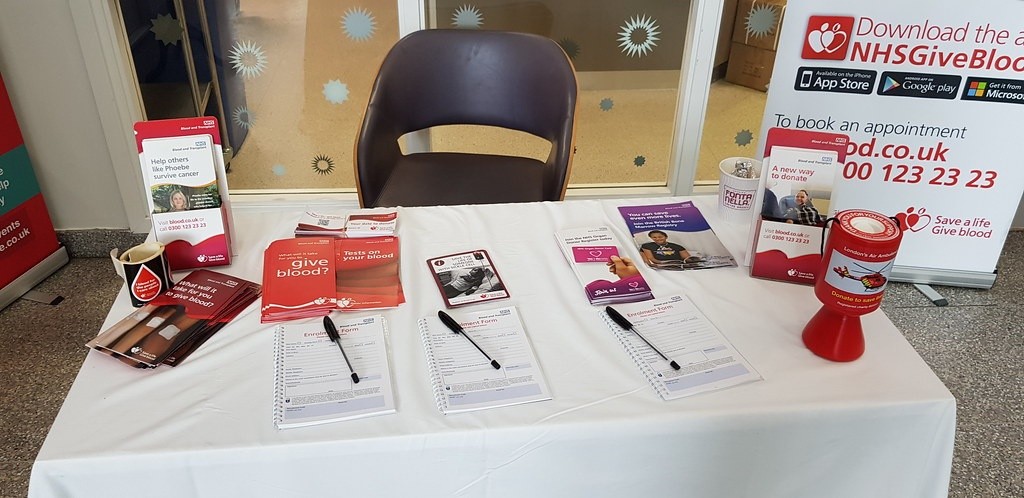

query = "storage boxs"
[725,0,788,92]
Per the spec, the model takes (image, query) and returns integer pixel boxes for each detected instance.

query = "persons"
[606,255,637,280]
[762,187,782,220]
[168,190,190,211]
[780,189,822,226]
[640,231,694,266]
[442,267,485,299]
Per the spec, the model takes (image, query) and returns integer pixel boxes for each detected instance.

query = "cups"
[718,156,763,224]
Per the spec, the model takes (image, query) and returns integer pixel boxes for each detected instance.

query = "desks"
[26,196,956,498]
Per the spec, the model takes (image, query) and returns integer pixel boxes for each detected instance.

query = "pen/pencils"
[323,315,360,384]
[606,306,681,370]
[437,309,502,370]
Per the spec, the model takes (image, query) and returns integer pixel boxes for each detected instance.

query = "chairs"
[353,28,580,210]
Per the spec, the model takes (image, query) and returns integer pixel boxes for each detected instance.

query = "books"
[273,313,398,430]
[599,294,764,400]
[417,305,553,414]
